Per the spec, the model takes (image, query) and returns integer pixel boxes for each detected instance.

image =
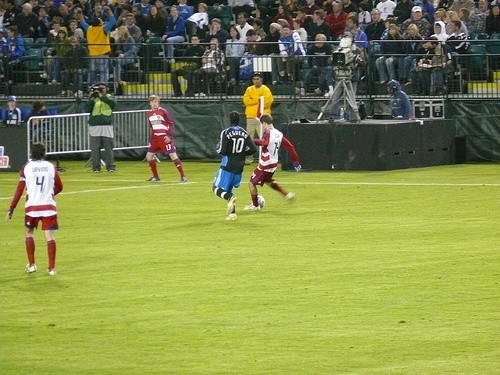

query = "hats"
[270,23,282,31]
[412,6,422,12]
[7,96,17,101]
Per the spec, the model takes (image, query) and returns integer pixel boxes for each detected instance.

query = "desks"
[0,126,31,171]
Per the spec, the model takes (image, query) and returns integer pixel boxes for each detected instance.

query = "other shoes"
[228,195,237,210]
[323,86,333,96]
[25,263,37,273]
[146,176,160,181]
[179,176,187,183]
[47,269,57,276]
[225,213,238,221]
[285,192,296,202]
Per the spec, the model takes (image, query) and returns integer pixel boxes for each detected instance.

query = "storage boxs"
[408,99,445,119]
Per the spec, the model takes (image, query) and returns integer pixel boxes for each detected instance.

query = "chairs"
[0,6,500,97]
[0,108,57,122]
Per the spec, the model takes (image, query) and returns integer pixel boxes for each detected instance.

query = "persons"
[1,95,22,128]
[145,94,187,182]
[0,0,500,100]
[212,109,258,220]
[25,101,51,128]
[329,31,365,124]
[6,142,64,276]
[244,114,303,210]
[242,73,275,165]
[385,80,413,120]
[84,83,116,173]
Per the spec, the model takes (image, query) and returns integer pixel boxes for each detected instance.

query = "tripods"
[314,75,362,124]
